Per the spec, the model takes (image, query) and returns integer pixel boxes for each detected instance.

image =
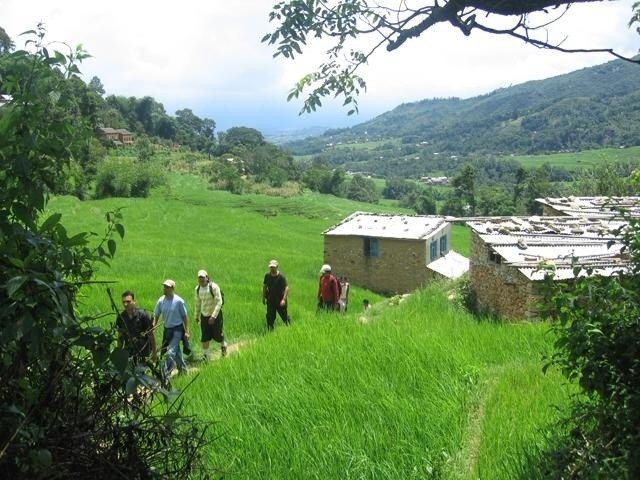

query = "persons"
[150,279,191,378]
[193,270,227,360]
[261,260,291,330]
[316,263,372,314]
[115,291,158,404]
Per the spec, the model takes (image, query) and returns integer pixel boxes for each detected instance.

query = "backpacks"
[208,281,225,307]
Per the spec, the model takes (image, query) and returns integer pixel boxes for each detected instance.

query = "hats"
[197,269,208,278]
[161,279,176,288]
[268,259,279,268]
[319,264,332,274]
